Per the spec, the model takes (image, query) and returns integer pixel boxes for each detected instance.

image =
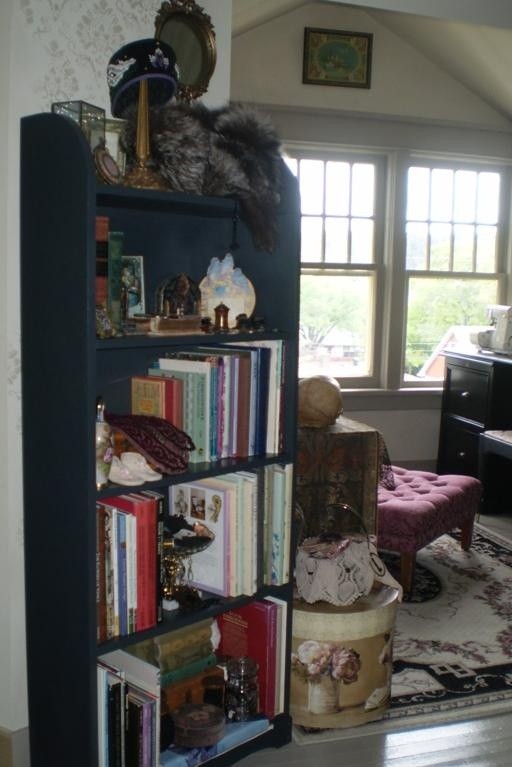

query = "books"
[128,616,217,686]
[96,215,145,327]
[131,338,286,463]
[96,491,165,646]
[214,595,287,718]
[170,462,294,599]
[97,648,161,764]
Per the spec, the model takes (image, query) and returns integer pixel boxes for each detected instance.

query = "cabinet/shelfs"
[17,108,300,766]
[433,346,511,514]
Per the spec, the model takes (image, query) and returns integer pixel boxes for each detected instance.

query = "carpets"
[284,523,511,748]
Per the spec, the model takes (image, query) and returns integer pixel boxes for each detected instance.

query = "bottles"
[223,659,262,725]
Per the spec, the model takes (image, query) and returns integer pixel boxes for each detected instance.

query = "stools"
[373,464,483,600]
[289,583,397,730]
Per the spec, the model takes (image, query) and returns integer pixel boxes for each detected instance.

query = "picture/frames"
[300,26,373,90]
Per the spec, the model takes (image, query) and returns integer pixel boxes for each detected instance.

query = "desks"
[300,412,382,552]
